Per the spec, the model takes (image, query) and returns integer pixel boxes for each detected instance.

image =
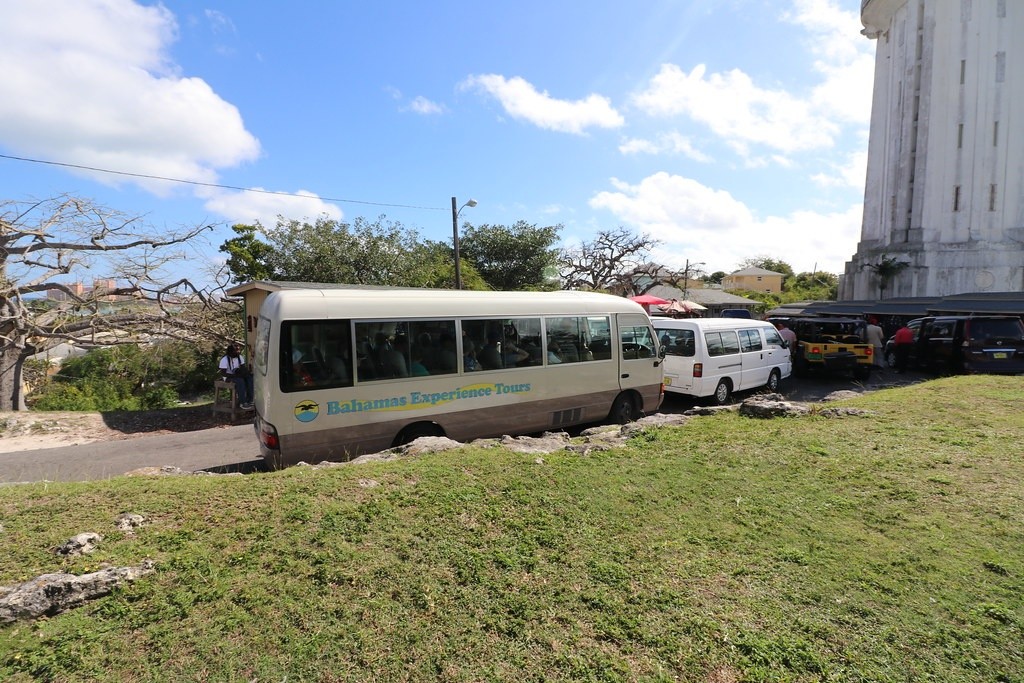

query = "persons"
[893,321,912,374]
[859,319,885,372]
[293,325,595,387]
[219,344,255,410]
[778,322,797,363]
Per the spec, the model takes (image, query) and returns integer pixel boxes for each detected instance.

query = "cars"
[720,309,752,320]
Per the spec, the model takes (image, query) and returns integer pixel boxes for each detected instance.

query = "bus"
[252,290,667,468]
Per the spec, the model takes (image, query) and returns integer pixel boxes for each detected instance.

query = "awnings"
[766,291,1024,317]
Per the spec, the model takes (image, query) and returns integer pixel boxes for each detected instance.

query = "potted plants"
[856,254,910,288]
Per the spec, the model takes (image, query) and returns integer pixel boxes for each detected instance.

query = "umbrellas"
[628,294,671,311]
[659,298,709,316]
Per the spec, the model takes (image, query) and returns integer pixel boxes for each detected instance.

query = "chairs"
[314,344,580,384]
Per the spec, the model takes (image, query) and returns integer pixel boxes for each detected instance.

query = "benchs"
[211,380,239,421]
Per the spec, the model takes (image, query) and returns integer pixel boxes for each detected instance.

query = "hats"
[869,318,877,324]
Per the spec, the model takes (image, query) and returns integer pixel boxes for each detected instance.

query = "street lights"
[683,259,706,300]
[452,197,477,290]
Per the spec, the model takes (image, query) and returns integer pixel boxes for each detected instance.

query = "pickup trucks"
[785,317,873,377]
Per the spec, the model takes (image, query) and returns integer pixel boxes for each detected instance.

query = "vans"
[571,317,674,342]
[653,318,793,404]
[885,315,1024,375]
